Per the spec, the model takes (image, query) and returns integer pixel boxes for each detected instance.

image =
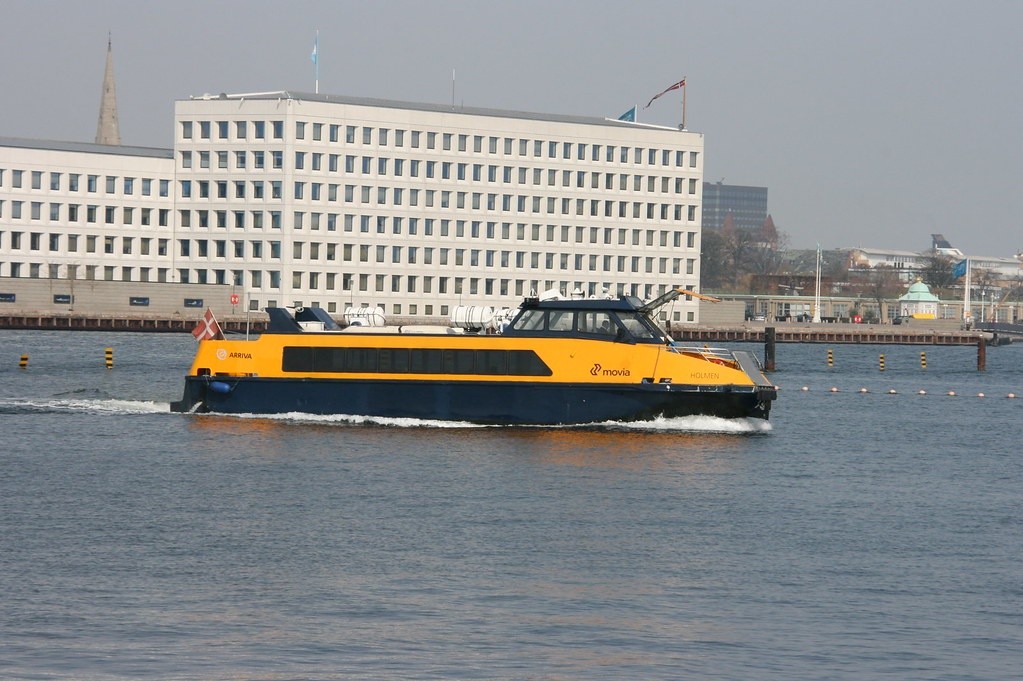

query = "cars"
[893,315,915,325]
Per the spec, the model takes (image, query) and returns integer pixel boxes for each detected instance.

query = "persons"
[599,320,609,334]
[803,312,807,319]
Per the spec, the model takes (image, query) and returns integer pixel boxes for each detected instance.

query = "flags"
[951,258,967,282]
[192,309,219,344]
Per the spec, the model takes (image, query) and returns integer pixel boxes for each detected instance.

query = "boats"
[169,289,778,430]
